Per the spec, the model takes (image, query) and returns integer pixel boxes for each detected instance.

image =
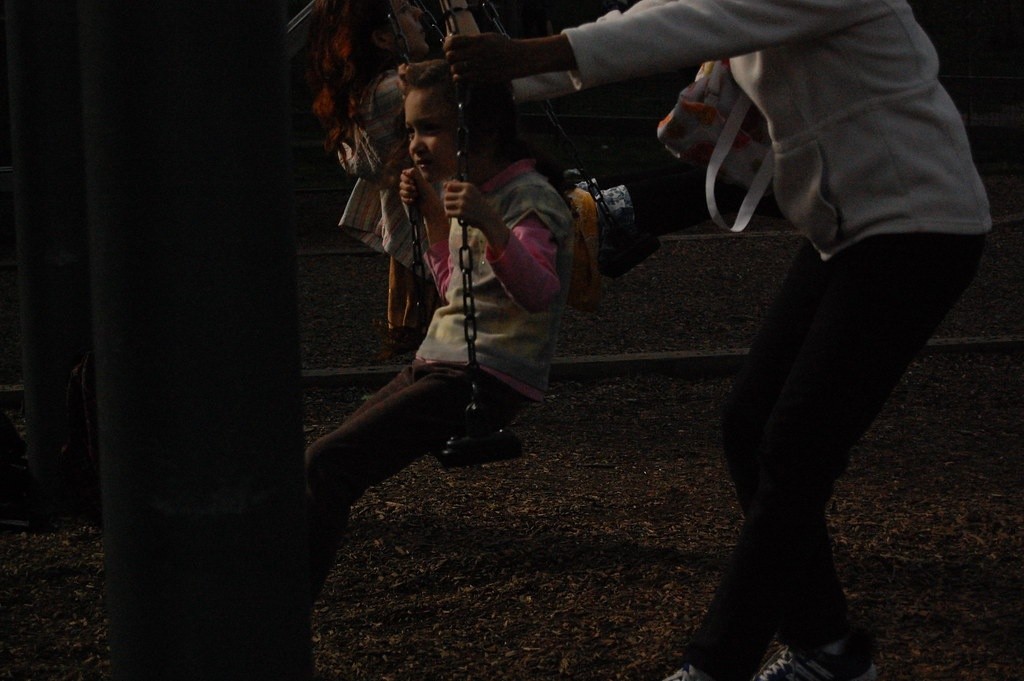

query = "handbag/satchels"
[658,59,776,235]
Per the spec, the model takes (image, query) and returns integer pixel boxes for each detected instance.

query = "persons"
[299,59,574,603]
[440,0,992,680]
[306,0,786,331]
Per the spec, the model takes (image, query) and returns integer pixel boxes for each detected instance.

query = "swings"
[414,0,661,278]
[377,0,524,470]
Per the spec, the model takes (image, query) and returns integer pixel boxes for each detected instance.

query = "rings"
[464,59,479,68]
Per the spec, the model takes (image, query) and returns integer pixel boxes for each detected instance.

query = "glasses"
[396,1,415,15]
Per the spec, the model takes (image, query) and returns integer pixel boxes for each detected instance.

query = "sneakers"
[663,665,710,681]
[753,630,877,681]
